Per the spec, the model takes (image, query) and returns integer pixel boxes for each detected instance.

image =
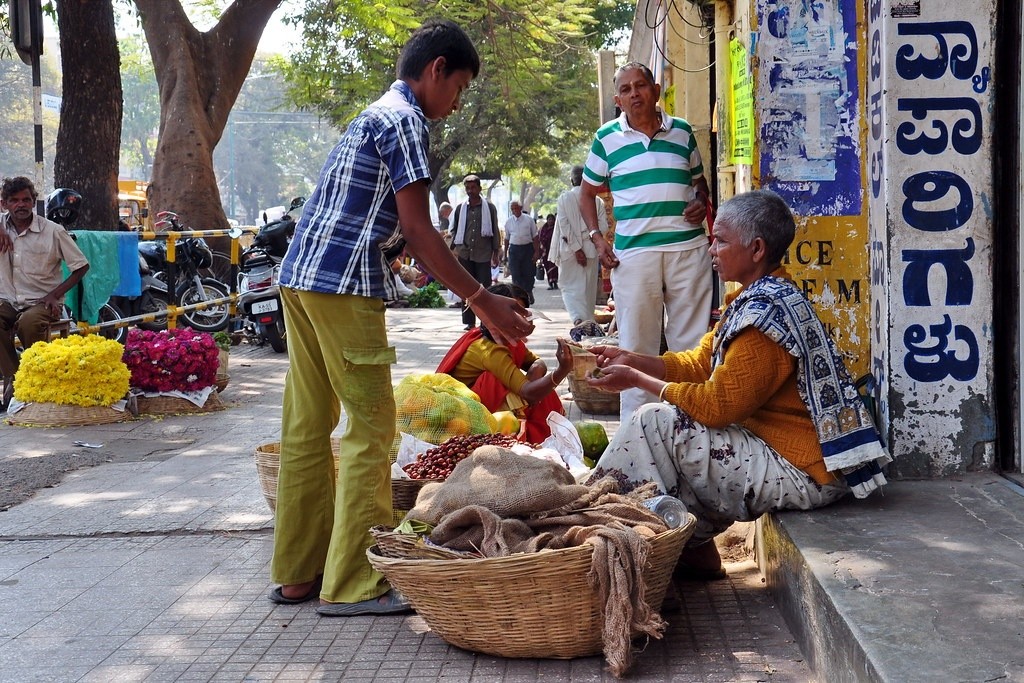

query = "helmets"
[45,188,83,224]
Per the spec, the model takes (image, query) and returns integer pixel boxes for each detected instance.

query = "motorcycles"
[43,189,231,344]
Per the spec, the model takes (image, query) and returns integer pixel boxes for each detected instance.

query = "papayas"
[490,410,519,437]
[573,422,609,468]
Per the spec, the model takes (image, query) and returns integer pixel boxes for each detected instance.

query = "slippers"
[268,581,323,605]
[316,588,414,616]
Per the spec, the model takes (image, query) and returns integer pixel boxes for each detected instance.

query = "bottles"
[641,495,688,530]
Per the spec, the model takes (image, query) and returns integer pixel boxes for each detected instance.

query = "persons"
[576,61,714,358]
[267,18,535,617]
[0,175,90,408]
[433,282,574,445]
[583,190,893,581]
[439,164,608,330]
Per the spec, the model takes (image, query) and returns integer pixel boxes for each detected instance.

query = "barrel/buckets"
[566,356,620,414]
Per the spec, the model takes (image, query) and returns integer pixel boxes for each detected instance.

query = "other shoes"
[530,293,535,304]
[463,325,476,331]
[547,284,559,290]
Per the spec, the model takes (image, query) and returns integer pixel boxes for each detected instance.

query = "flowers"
[12,326,220,408]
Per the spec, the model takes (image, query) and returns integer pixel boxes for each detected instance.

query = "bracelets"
[463,284,484,313]
[659,381,672,402]
[550,371,560,386]
[588,229,603,242]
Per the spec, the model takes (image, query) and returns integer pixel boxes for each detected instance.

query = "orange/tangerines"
[390,387,496,459]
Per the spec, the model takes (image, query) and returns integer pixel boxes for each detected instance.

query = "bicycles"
[150,210,239,288]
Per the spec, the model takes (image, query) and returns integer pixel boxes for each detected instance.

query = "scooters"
[229,196,308,353]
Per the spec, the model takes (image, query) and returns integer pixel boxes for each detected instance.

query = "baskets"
[556,336,620,416]
[254,438,345,512]
[388,478,439,512]
[365,501,699,658]
